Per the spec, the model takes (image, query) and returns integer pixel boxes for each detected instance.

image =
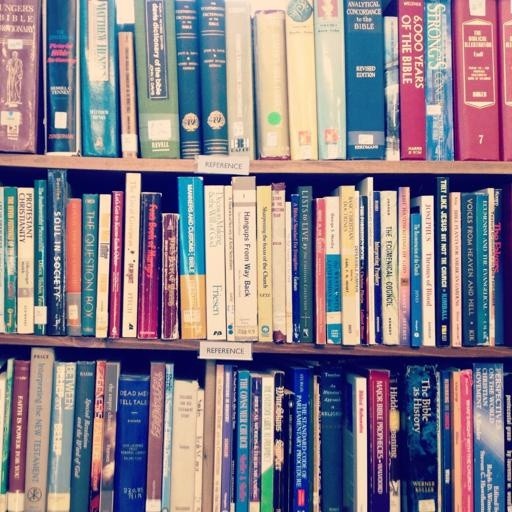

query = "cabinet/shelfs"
[0,1,511,512]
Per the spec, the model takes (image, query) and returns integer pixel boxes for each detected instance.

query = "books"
[1,0,511,161]
[0,348,512,512]
[1,172,512,348]
